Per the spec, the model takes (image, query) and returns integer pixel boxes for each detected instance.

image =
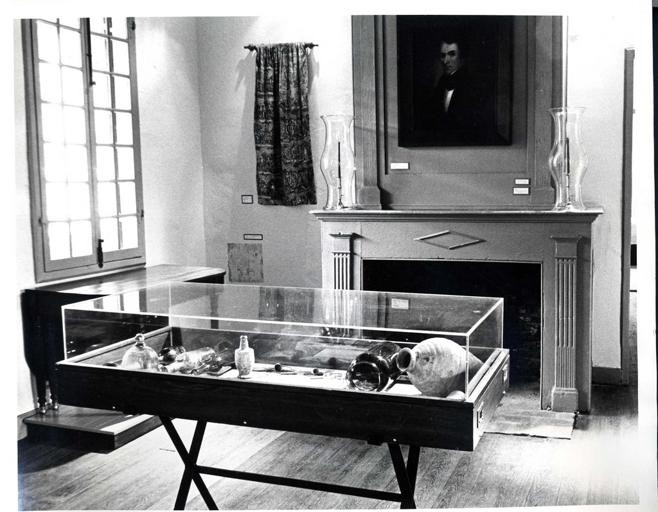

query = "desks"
[21,264,226,414]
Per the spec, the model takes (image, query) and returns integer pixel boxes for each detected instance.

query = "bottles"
[156,334,255,379]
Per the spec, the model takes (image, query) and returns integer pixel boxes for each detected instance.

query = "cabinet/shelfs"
[55,280,511,510]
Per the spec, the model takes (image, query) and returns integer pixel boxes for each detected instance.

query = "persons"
[420,37,495,146]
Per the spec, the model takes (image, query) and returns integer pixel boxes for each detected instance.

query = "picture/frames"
[396,14,515,147]
